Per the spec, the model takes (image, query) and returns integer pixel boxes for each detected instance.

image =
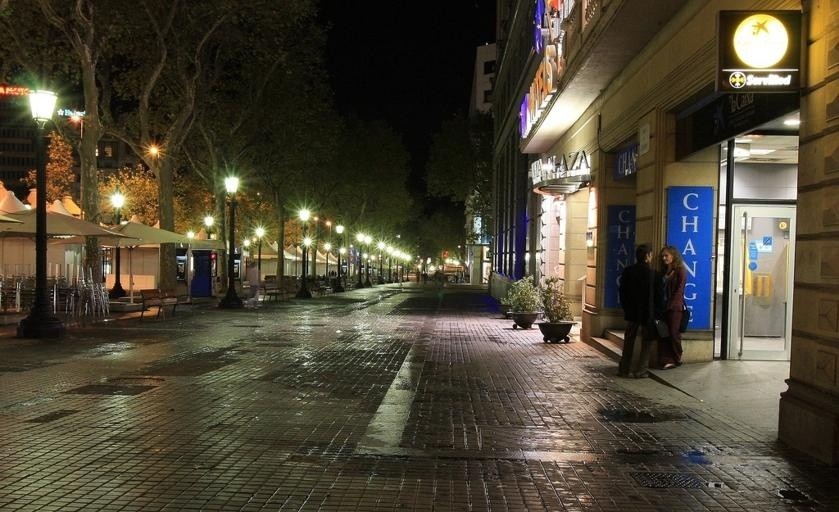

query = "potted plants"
[536,272,578,346]
[497,273,545,330]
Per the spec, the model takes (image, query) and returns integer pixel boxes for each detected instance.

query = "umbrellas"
[51,220,194,304]
[0,205,141,240]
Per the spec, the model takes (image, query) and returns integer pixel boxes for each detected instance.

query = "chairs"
[1,258,115,325]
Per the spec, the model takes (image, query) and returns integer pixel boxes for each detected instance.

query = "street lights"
[253,226,263,282]
[332,223,344,293]
[204,215,213,239]
[324,241,331,275]
[355,230,414,288]
[295,205,315,300]
[303,237,311,277]
[104,183,131,298]
[216,171,246,312]
[10,86,69,340]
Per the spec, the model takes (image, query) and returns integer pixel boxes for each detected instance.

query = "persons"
[617,243,660,379]
[416,271,432,283]
[454,265,468,283]
[656,245,688,369]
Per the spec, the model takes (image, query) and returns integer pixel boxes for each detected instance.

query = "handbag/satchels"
[679,310,690,333]
[647,318,670,340]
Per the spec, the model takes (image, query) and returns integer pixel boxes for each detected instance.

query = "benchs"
[262,283,291,306]
[139,289,180,325]
[310,280,332,298]
